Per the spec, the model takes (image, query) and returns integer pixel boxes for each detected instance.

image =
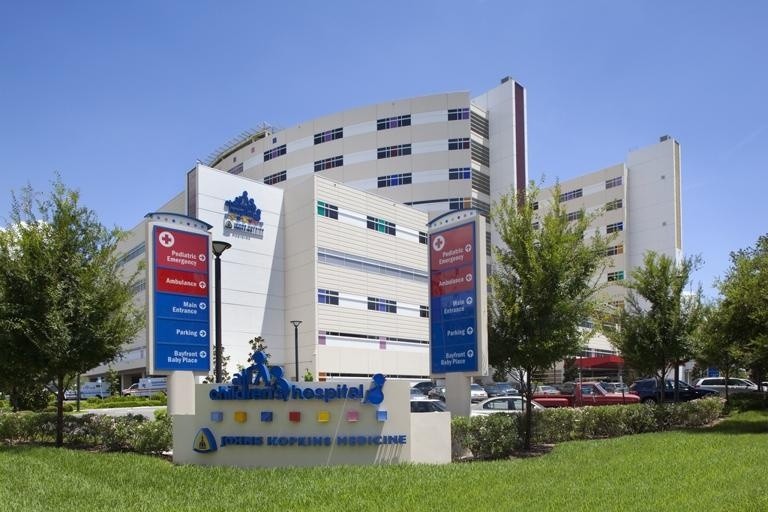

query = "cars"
[122,383,160,397]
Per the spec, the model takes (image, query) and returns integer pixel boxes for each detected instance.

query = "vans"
[80,381,111,400]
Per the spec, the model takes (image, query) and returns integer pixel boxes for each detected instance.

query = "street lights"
[290,320,303,382]
[211,240,232,383]
